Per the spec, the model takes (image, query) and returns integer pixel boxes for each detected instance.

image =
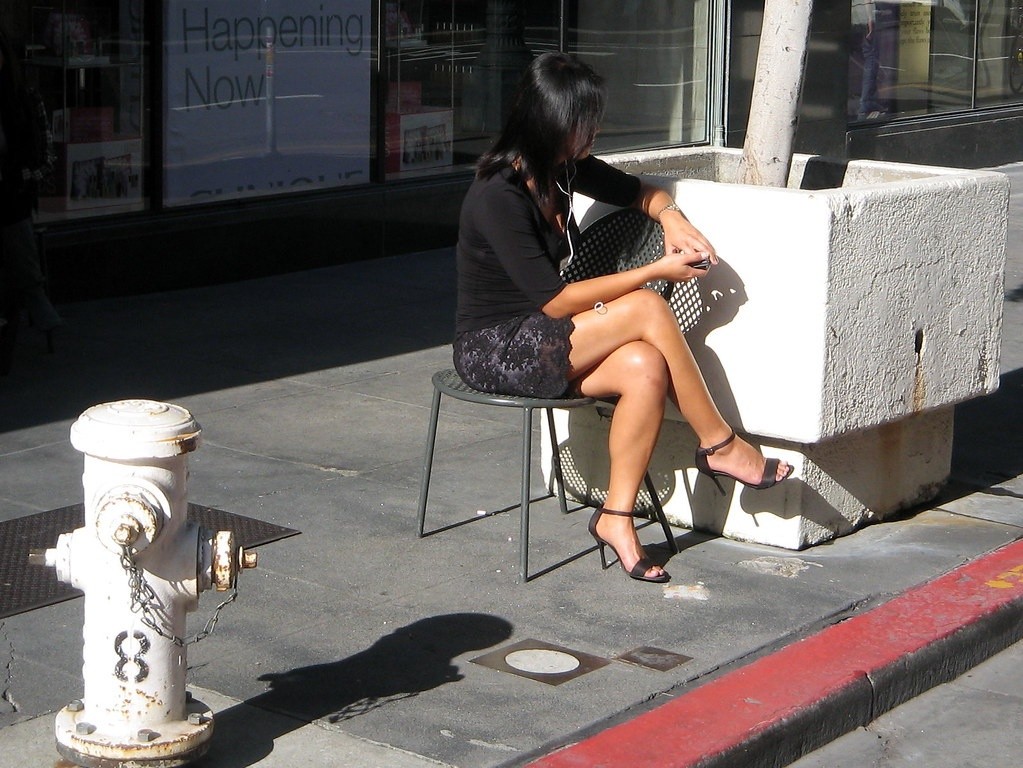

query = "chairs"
[416,208,678,583]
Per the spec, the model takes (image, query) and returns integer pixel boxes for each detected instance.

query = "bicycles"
[1006,5,1023,96]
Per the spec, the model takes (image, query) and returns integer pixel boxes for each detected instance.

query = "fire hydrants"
[26,397,259,768]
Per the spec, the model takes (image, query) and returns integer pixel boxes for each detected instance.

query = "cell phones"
[688,259,709,271]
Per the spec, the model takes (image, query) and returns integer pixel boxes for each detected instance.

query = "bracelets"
[657,203,680,223]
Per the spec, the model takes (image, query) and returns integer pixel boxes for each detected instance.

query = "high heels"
[587,504,669,582]
[694,431,794,497]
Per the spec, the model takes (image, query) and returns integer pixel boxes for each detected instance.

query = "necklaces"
[548,184,556,191]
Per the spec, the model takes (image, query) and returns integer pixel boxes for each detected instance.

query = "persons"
[47,65,120,197]
[452,50,792,583]
[0,26,64,378]
[405,127,448,163]
[851,0,888,115]
